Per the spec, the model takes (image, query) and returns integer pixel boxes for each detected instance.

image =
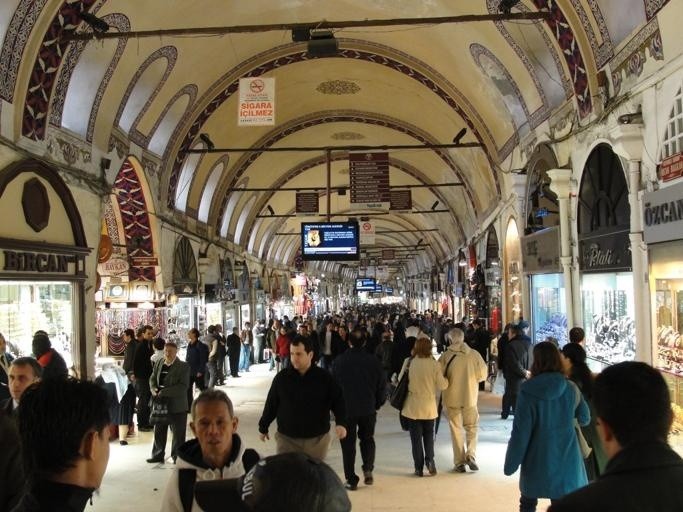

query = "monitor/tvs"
[301,222,360,261]
[368,285,382,293]
[384,287,393,292]
[355,278,376,291]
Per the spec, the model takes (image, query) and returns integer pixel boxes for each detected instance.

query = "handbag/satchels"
[573,418,592,459]
[390,374,408,410]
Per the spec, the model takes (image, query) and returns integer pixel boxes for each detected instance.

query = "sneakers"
[415,469,423,476]
[428,463,436,474]
[465,456,478,470]
[147,459,163,462]
[454,465,466,472]
[365,470,373,484]
[344,481,356,490]
